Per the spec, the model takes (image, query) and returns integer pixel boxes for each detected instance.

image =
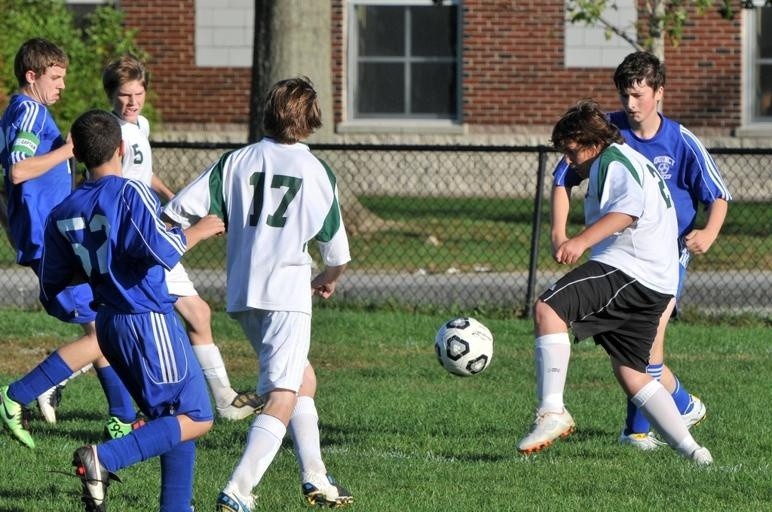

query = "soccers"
[434,317,494,378]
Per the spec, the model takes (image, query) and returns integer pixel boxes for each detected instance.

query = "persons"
[0,37,148,451]
[159,77,354,512]
[549,52,731,450]
[39,108,214,512]
[36,58,256,423]
[517,104,713,468]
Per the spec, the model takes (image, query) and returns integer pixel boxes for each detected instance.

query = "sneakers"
[681,393,707,430]
[216,481,258,512]
[691,446,713,468]
[301,470,354,508]
[36,379,66,424]
[72,443,108,512]
[516,406,575,453]
[104,410,147,440]
[0,384,35,448]
[215,385,265,422]
[620,427,668,450]
